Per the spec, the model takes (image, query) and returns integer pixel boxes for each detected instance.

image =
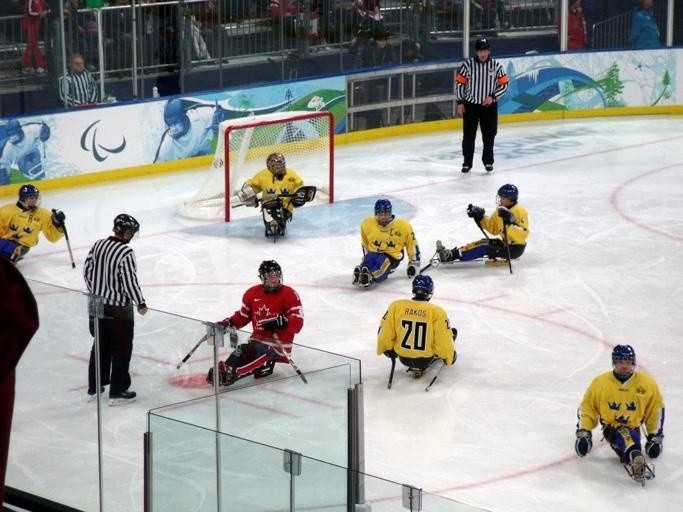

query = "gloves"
[214,318,233,335]
[383,348,397,358]
[408,262,423,276]
[50,209,65,228]
[246,197,260,208]
[497,206,514,226]
[259,316,288,332]
[574,429,593,457]
[644,432,663,458]
[466,205,484,221]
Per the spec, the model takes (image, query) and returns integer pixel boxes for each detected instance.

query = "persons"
[0,118,49,186]
[82,214,149,407]
[350,198,421,288]
[237,152,305,237]
[376,275,457,369]
[436,182,529,263]
[574,345,665,487]
[454,38,509,175]
[627,0,661,49]
[557,0,589,52]
[1,184,69,265]
[153,96,226,165]
[205,260,304,386]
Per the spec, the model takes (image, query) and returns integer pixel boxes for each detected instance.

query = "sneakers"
[353,267,361,285]
[108,388,137,400]
[267,201,289,229]
[214,360,233,386]
[361,266,370,288]
[87,382,106,395]
[484,163,493,172]
[436,240,458,264]
[461,165,471,174]
[629,451,644,482]
[449,326,458,344]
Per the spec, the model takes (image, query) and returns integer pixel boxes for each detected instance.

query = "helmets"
[258,259,282,289]
[112,214,139,237]
[374,200,391,224]
[412,275,434,301]
[18,184,41,209]
[163,99,189,137]
[265,152,286,178]
[495,184,518,206]
[611,343,635,379]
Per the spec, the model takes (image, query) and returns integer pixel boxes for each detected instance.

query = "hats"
[476,38,490,50]
